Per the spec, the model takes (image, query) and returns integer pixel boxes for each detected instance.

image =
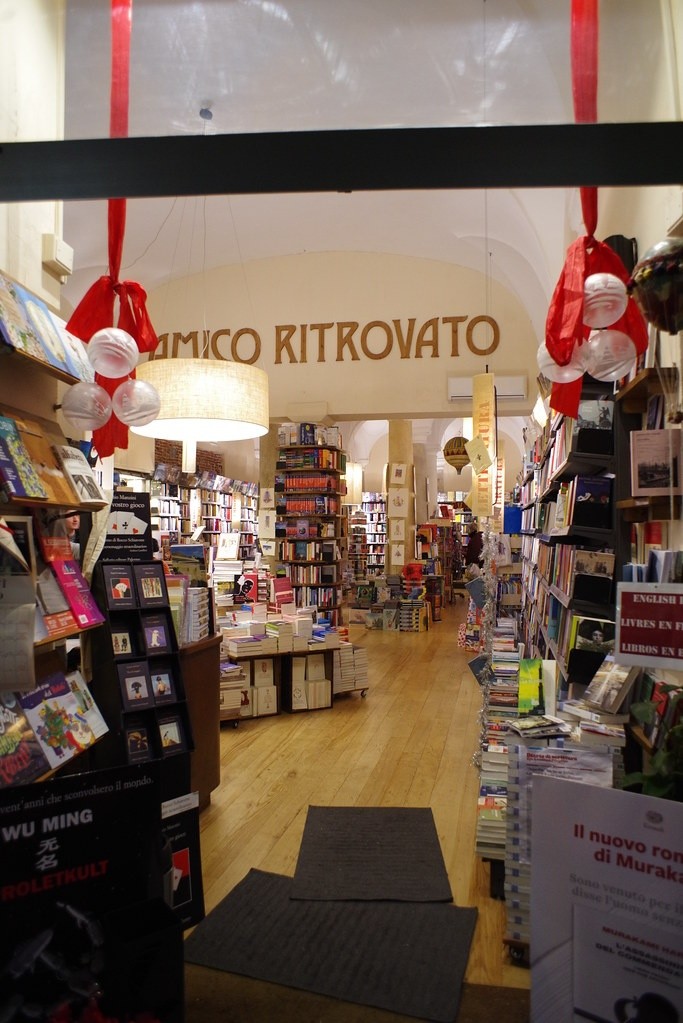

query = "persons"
[65,509,80,544]
[152,538,171,574]
[465,522,484,571]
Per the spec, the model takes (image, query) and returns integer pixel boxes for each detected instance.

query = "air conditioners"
[447,374,528,402]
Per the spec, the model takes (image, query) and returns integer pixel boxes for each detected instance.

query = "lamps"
[340,462,362,505]
[128,358,270,441]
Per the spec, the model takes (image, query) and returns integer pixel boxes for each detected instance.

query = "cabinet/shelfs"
[0,274,683,1023]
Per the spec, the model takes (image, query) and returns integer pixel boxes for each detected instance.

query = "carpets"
[184,868,480,1023]
[289,804,455,905]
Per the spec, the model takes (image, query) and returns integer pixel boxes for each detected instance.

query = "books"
[438,490,472,566]
[163,544,209,645]
[209,533,349,657]
[332,640,370,693]
[102,562,189,764]
[273,422,347,538]
[359,492,386,574]
[218,653,277,719]
[435,526,454,606]
[0,273,109,792]
[472,372,682,946]
[144,462,260,547]
[347,517,368,576]
[288,654,332,709]
